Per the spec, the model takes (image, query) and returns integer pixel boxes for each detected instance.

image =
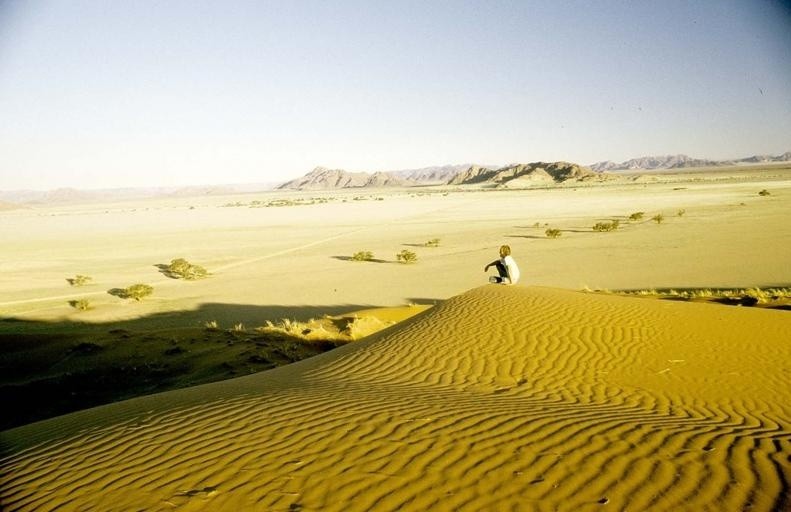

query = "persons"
[483,243,519,286]
